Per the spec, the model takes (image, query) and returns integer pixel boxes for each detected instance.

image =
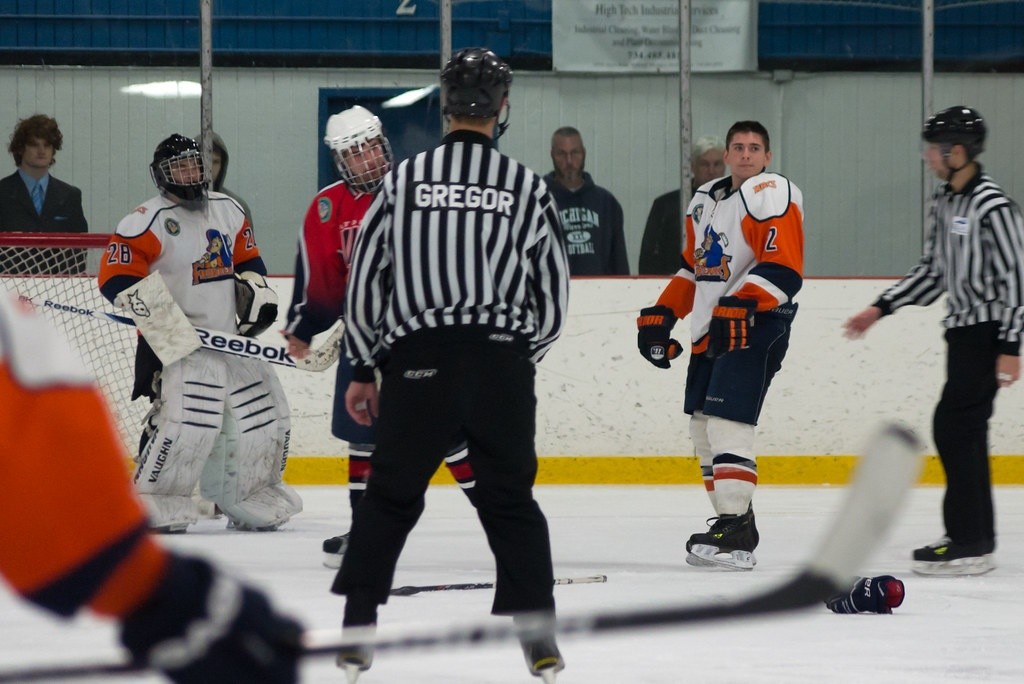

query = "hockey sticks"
[3,422,927,684]
[388,574,607,597]
[18,293,346,372]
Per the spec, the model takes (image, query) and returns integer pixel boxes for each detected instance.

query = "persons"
[639,133,726,275]
[0,112,89,274]
[0,282,307,684]
[195,129,253,228]
[636,120,805,572]
[543,127,631,276]
[843,105,1024,576]
[330,47,572,684]
[279,102,478,567]
[97,134,304,534]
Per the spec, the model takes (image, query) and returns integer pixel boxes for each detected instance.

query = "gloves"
[706,297,757,358]
[638,305,683,369]
[122,553,305,683]
[825,575,906,615]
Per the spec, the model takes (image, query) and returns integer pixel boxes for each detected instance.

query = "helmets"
[441,48,513,119]
[921,105,985,142]
[149,133,212,200]
[324,105,395,193]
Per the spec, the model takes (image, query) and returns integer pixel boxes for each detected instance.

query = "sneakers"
[912,533,996,578]
[337,611,378,684]
[686,499,759,569]
[322,532,350,569]
[515,610,565,683]
